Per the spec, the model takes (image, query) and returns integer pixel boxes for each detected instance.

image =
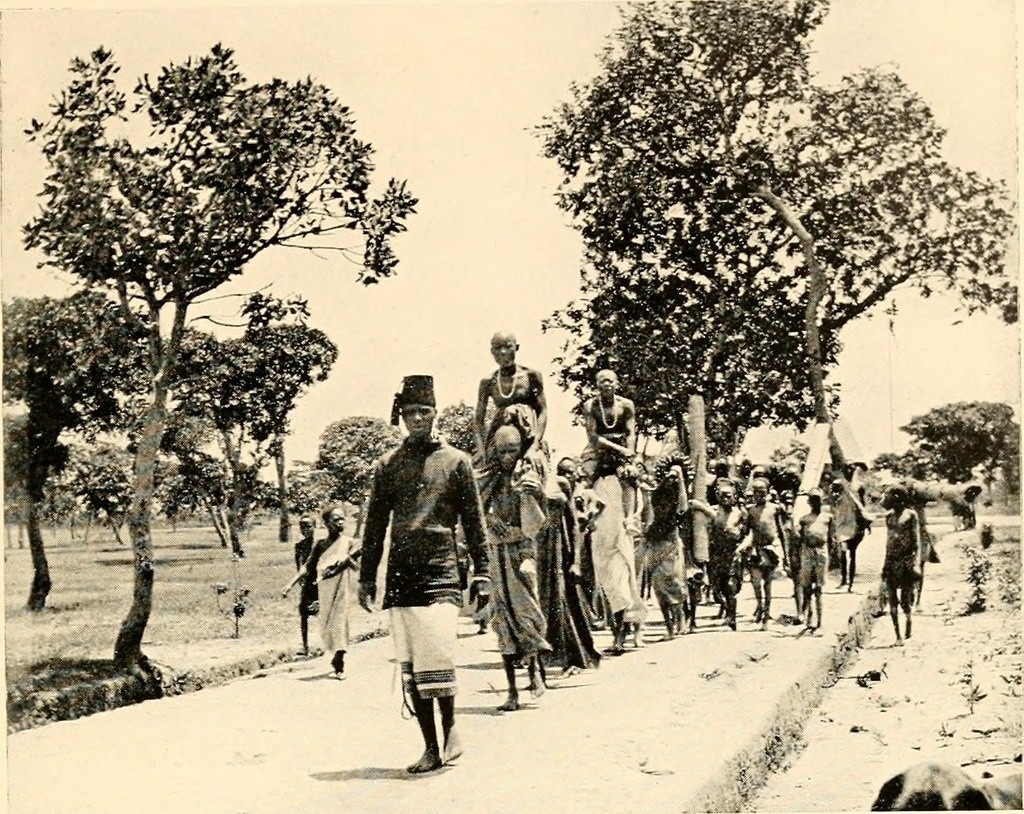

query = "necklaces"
[599,398,618,428]
[498,368,518,397]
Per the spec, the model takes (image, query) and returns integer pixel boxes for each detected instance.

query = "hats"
[391,375,435,425]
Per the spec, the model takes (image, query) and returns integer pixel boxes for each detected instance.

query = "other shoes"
[328,670,345,680]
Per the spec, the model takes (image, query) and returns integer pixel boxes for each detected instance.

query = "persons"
[358,374,492,772]
[454,329,874,711]
[902,487,940,612]
[294,515,318,656]
[907,487,921,506]
[282,506,361,680]
[881,485,922,647]
